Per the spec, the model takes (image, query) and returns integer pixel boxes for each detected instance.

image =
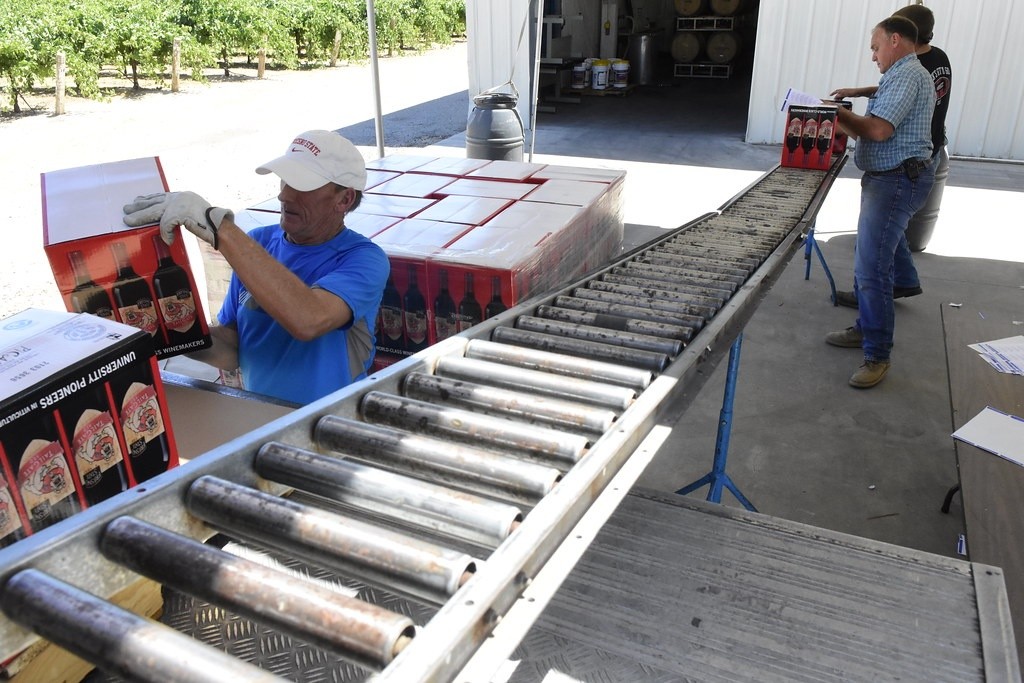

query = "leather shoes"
[831,289,860,308]
[892,283,923,299]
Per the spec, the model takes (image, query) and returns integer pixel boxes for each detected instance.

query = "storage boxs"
[821,98,853,155]
[0,308,179,677]
[39,155,212,362]
[781,103,838,170]
[196,151,627,371]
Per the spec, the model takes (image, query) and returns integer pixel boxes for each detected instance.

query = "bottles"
[0,359,170,549]
[373,263,506,353]
[834,133,842,147]
[67,235,204,351]
[786,112,835,165]
[517,204,609,307]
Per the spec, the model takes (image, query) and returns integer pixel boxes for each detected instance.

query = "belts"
[869,158,934,176]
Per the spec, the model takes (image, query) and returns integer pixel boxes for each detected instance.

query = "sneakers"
[824,323,864,347]
[848,360,891,386]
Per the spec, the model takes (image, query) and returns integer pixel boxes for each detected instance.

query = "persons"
[123,129,390,405]
[819,4,953,389]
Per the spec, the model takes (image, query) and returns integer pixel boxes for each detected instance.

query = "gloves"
[124,190,234,250]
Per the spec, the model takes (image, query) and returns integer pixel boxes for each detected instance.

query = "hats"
[255,129,367,192]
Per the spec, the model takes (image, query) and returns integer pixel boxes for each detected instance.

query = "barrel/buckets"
[706,31,743,63]
[671,32,706,62]
[466,92,525,163]
[572,57,630,89]
[709,0,748,16]
[673,0,707,17]
